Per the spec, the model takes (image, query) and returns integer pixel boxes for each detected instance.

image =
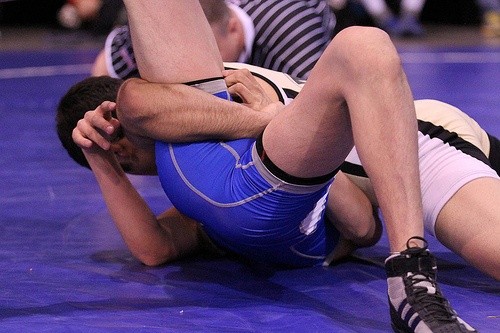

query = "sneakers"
[385,246,477,333]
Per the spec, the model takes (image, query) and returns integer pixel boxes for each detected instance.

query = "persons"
[89,0,385,83]
[72,0,479,333]
[56,59,500,282]
